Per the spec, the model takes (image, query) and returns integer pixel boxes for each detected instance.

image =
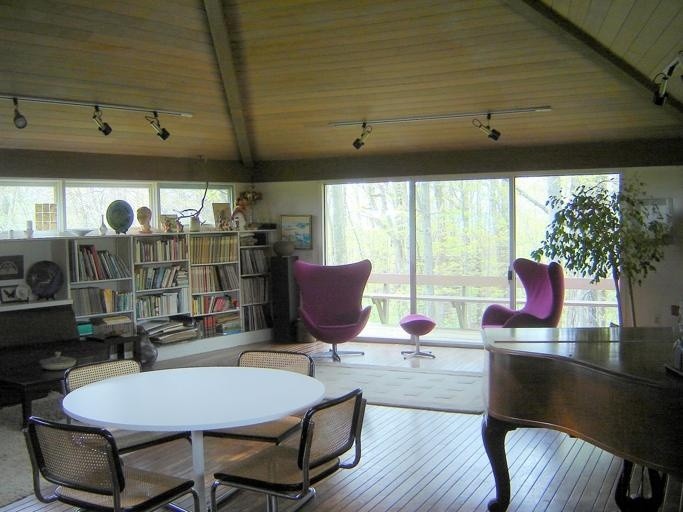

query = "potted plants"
[0,284,19,304]
[0,252,25,281]
[278,213,313,251]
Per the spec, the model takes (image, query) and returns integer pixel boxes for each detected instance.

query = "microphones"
[391,310,441,360]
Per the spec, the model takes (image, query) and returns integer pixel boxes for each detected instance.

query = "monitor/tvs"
[70,233,274,344]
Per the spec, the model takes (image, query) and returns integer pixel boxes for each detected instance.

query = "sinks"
[0,303,127,430]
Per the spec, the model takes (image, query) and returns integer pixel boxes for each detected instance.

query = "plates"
[0,229,278,362]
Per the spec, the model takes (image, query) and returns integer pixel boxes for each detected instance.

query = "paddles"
[271,357,487,418]
[0,388,155,511]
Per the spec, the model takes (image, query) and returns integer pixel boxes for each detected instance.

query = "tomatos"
[481,325,683,512]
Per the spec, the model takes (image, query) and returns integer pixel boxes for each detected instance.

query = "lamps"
[208,385,366,512]
[203,349,313,446]
[56,356,193,466]
[18,412,203,511]
[289,257,377,366]
[482,257,567,332]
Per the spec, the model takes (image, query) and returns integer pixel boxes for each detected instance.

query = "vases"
[232,184,264,209]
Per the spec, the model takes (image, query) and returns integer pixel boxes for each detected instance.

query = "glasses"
[0,91,197,143]
[646,52,682,107]
[323,100,556,152]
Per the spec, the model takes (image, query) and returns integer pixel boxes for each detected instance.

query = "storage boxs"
[354,289,620,333]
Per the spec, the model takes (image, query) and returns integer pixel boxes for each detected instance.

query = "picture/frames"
[87,331,141,362]
[59,364,329,511]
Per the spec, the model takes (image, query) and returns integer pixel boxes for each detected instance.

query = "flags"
[97,214,108,236]
[273,239,296,259]
[134,331,160,372]
[244,205,253,226]
